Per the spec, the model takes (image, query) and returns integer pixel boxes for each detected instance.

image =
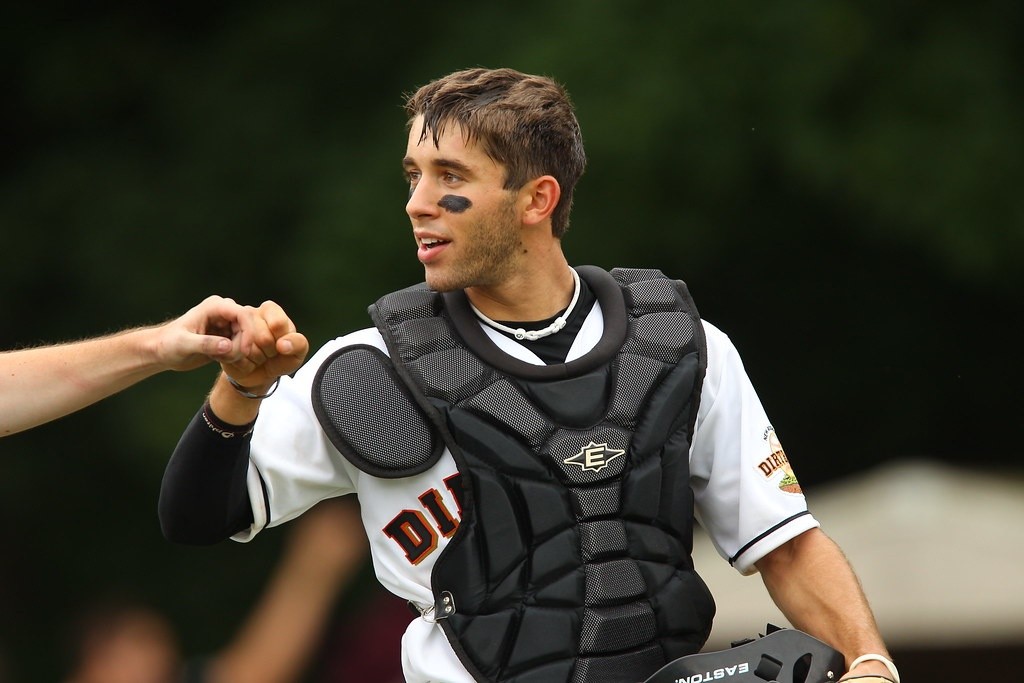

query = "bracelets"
[232,379,283,400]
[838,654,902,683]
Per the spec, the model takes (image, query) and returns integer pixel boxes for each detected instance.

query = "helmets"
[640,628,846,683]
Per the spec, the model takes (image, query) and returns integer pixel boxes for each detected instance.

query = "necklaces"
[466,261,582,340]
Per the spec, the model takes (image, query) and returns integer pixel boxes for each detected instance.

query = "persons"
[157,67,901,683]
[0,293,256,438]
[66,502,367,683]
